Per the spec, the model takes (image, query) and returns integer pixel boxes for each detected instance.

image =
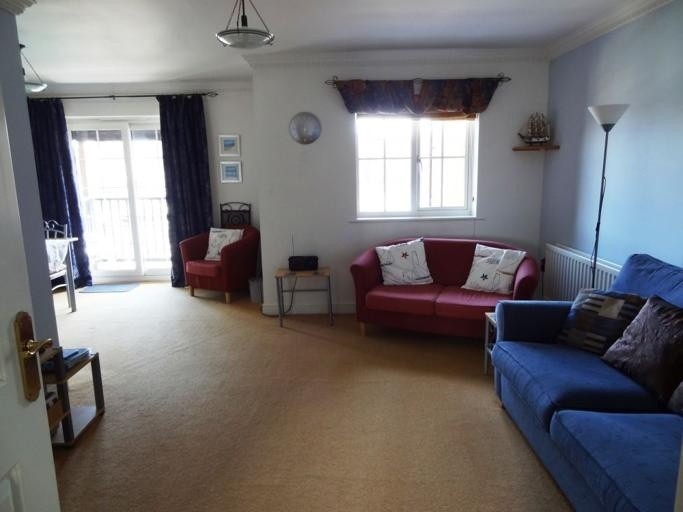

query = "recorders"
[288,255,318,271]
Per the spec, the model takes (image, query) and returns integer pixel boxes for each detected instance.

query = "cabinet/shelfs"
[41,344,105,449]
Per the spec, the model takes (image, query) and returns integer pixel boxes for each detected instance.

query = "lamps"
[215,0,274,49]
[19,43,47,94]
[587,103,631,289]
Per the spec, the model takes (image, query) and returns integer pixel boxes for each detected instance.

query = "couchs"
[349,237,539,337]
[489,299,682,512]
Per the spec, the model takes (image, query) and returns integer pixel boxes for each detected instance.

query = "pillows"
[606,253,683,309]
[554,288,649,356]
[600,293,683,406]
[375,236,434,286]
[203,227,245,261]
[666,382,683,417]
[460,243,527,295]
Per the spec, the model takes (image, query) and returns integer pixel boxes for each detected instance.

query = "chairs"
[43,219,71,307]
[179,224,259,304]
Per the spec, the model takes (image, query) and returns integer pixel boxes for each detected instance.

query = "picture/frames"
[220,161,242,183]
[219,134,240,157]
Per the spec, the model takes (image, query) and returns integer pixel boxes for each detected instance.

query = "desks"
[274,266,334,327]
[45,237,78,312]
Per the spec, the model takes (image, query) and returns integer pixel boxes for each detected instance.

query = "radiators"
[542,241,623,300]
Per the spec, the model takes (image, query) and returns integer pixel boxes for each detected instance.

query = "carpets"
[79,282,141,293]
